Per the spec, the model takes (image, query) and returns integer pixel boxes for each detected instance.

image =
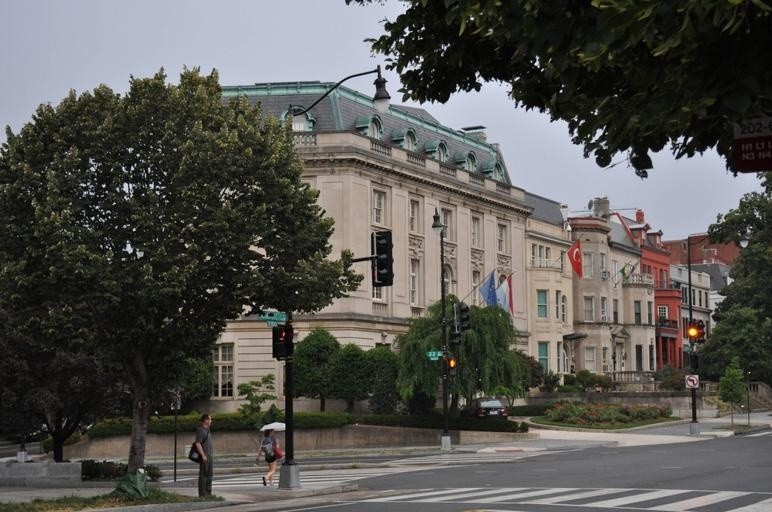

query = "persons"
[255,430,279,487]
[196,415,213,499]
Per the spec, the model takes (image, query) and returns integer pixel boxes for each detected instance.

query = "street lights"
[281,65,390,464]
[687,223,749,423]
[431,208,451,433]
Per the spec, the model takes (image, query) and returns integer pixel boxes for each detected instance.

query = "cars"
[461,396,508,419]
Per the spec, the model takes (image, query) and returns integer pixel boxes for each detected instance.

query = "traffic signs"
[425,352,442,357]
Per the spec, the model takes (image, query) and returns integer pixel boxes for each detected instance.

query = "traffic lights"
[451,331,461,345]
[688,320,705,345]
[271,325,289,357]
[448,357,456,368]
[460,305,470,330]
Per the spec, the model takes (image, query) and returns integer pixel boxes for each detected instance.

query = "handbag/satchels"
[189,442,203,463]
[273,448,284,459]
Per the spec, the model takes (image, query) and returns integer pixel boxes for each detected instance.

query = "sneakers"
[262,476,267,485]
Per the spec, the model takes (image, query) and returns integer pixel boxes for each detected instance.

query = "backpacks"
[261,438,275,458]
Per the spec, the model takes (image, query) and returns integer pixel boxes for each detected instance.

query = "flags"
[495,274,514,316]
[479,272,497,305]
[620,263,632,287]
[567,239,582,280]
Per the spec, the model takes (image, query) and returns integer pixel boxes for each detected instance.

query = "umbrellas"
[260,422,286,432]
